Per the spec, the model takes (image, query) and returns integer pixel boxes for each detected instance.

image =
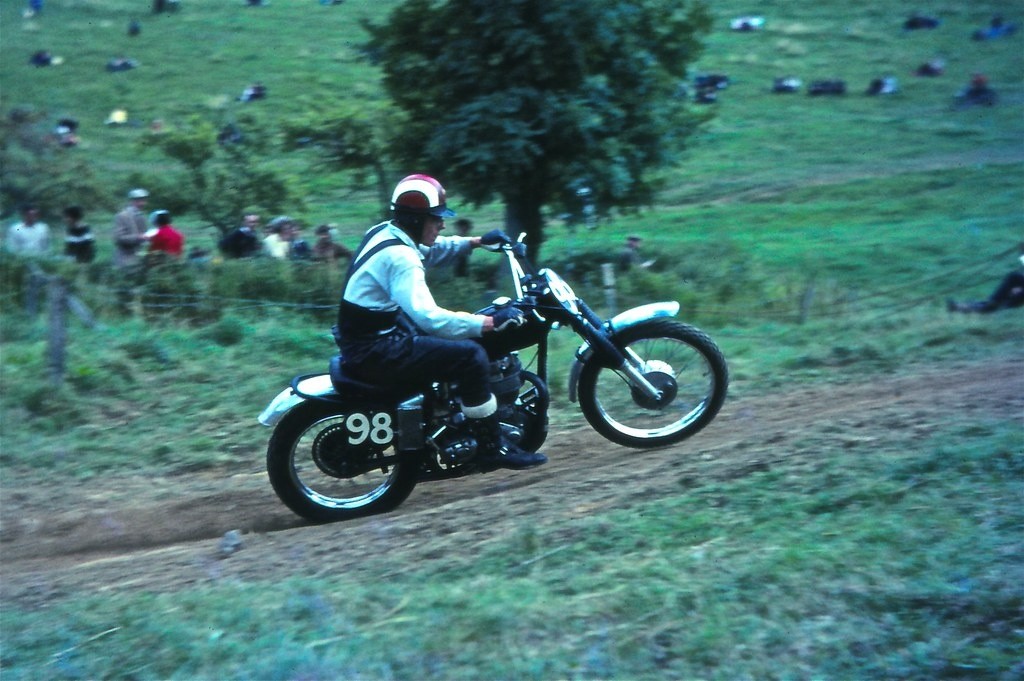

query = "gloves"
[492,308,528,331]
[481,229,512,253]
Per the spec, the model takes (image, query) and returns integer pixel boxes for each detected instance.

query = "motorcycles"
[257,232,729,526]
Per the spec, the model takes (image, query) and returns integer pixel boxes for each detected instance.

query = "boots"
[460,392,547,473]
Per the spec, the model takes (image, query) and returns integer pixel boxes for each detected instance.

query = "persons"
[945,255,1024,313]
[338,171,547,470]
[447,217,473,277]
[142,209,183,255]
[260,215,352,261]
[216,212,264,259]
[618,237,644,267]
[8,201,53,257]
[109,188,153,266]
[60,206,100,265]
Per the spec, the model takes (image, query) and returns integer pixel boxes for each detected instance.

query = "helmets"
[389,174,457,219]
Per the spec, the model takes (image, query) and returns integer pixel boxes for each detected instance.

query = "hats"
[315,225,335,234]
[128,189,150,199]
